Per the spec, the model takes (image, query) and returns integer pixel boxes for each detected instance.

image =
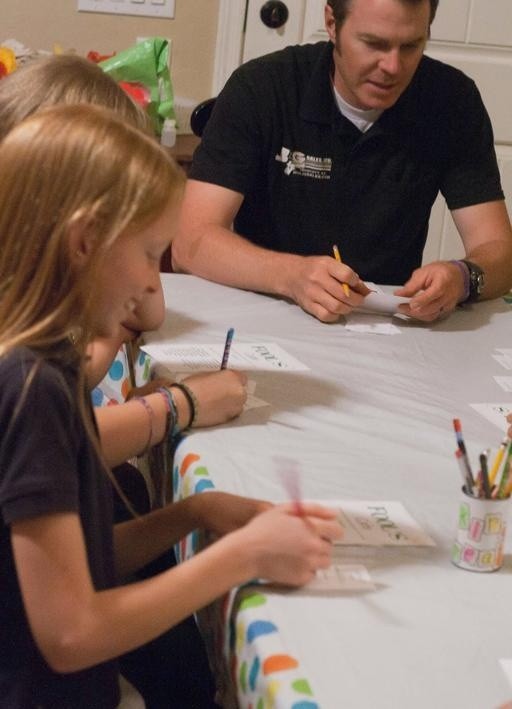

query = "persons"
[0,49,252,524]
[1,102,344,709]
[172,0,509,326]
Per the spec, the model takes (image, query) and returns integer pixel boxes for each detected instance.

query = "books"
[267,494,437,563]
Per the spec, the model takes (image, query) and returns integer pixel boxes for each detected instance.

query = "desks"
[86,266,512,709]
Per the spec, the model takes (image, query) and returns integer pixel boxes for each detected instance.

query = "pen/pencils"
[274,457,304,517]
[332,244,351,298]
[220,328,234,371]
[453,412,512,499]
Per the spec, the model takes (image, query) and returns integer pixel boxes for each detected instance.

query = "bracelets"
[170,379,201,438]
[449,257,470,305]
[153,384,180,447]
[129,393,154,462]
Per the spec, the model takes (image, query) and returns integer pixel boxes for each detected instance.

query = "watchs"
[459,259,486,305]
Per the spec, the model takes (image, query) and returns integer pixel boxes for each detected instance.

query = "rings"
[439,307,444,318]
